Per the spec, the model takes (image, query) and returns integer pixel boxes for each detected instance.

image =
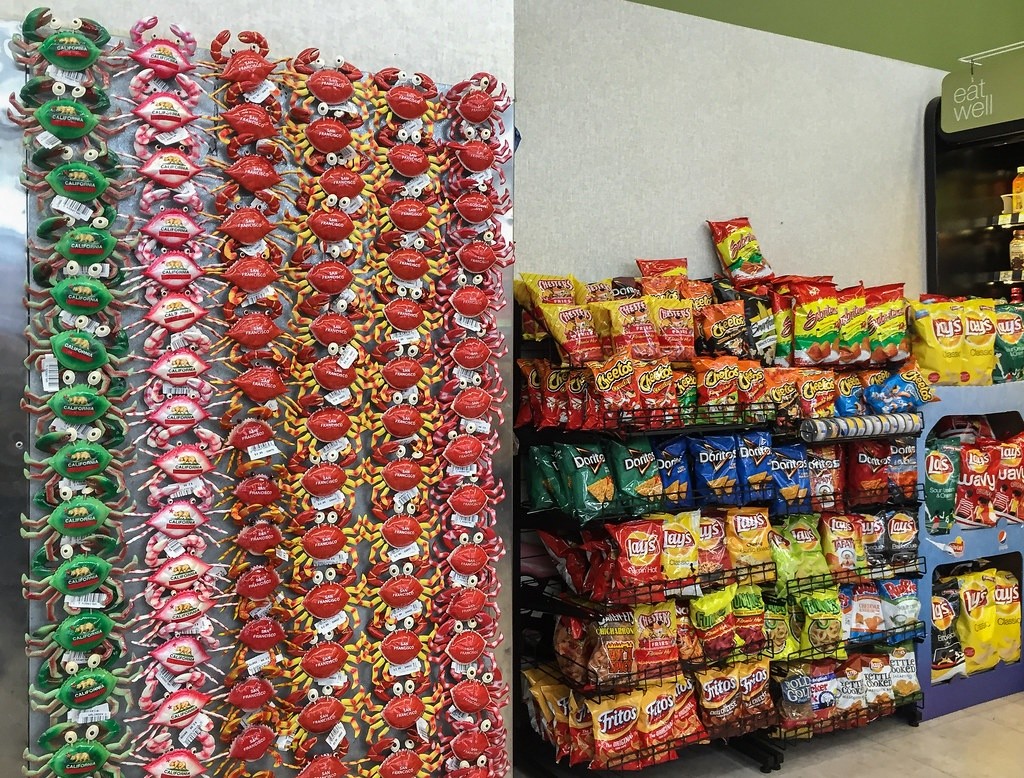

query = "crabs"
[7,5,516,778]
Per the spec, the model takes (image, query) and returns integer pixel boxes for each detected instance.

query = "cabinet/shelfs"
[924,96,1024,305]
[917,380,1024,721]
[513,301,928,777]
